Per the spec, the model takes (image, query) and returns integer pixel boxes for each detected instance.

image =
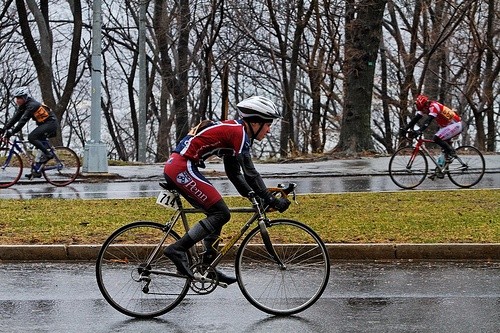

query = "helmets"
[236,95,282,122]
[416,96,428,110]
[14,86,28,97]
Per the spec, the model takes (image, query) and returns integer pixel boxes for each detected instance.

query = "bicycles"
[93,178,331,320]
[387,126,487,190]
[0,127,81,190]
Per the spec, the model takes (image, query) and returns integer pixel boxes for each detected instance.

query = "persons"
[0,90,59,177]
[401,96,463,168]
[163,97,291,285]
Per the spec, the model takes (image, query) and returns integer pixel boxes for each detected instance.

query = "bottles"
[437,153,442,164]
[35,149,41,162]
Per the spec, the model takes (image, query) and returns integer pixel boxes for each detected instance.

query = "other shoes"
[446,155,455,163]
[200,251,237,284]
[39,152,53,163]
[163,232,196,279]
[25,172,42,178]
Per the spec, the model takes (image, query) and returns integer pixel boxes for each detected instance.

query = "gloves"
[6,131,12,138]
[272,197,291,213]
[401,128,406,135]
[410,130,420,136]
